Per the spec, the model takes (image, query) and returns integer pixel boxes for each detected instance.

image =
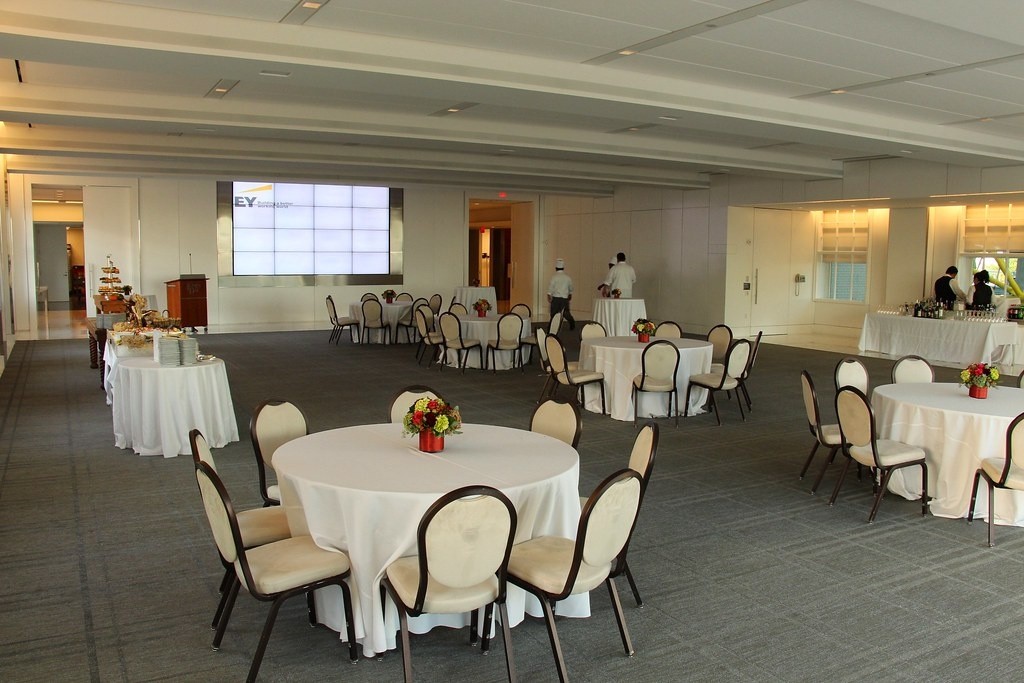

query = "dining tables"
[103,327,200,405]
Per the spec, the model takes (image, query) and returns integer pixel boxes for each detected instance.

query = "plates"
[153,334,197,367]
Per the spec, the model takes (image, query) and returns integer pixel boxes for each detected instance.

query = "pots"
[96,312,127,329]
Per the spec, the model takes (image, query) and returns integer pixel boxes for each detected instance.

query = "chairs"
[189,293,1024,683]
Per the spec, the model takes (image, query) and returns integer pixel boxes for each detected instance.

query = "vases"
[419,428,444,452]
[638,333,649,342]
[614,294,619,298]
[577,336,714,421]
[969,384,988,399]
[386,297,392,303]
[478,310,486,317]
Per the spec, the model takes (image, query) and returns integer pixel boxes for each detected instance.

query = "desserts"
[99,267,121,290]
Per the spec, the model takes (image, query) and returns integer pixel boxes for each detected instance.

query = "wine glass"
[955,309,1006,323]
[877,305,904,315]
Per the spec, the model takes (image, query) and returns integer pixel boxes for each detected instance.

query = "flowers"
[960,362,999,386]
[382,288,656,438]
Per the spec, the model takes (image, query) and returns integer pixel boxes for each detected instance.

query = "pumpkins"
[109,294,124,301]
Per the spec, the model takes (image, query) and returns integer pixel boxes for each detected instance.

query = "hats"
[556,258,564,268]
[609,256,617,265]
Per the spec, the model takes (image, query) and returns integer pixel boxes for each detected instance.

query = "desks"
[270,422,591,658]
[349,300,422,345]
[858,312,1017,370]
[454,286,497,315]
[113,354,240,459]
[871,383,1024,527]
[434,314,532,370]
[592,298,648,337]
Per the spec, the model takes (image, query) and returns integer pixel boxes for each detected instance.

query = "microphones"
[189,254,192,273]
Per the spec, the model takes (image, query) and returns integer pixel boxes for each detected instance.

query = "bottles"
[971,304,995,316]
[1007,303,1024,319]
[901,297,953,319]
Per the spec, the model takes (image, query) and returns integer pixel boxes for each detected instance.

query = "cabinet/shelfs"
[85,294,135,390]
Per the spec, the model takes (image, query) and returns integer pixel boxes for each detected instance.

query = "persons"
[602,256,617,297]
[935,266,966,309]
[966,270,996,311]
[598,252,636,298]
[547,259,576,330]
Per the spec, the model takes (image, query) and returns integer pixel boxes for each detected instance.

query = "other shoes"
[570,324,575,330]
